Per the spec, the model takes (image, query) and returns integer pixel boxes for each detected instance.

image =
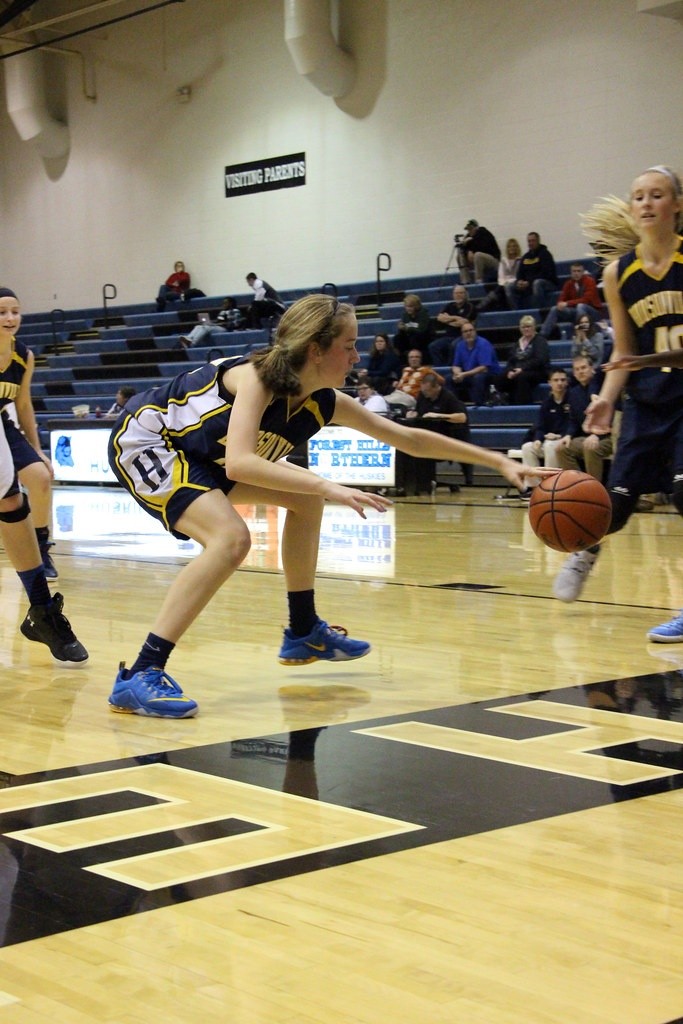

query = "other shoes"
[179,334,193,348]
[521,489,535,501]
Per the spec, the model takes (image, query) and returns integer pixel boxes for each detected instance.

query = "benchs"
[11,256,611,447]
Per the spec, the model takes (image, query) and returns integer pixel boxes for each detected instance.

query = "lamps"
[176,86,191,101]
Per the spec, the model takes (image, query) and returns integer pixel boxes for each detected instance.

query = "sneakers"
[555,544,601,603]
[279,619,373,666]
[36,540,58,580]
[108,661,201,720]
[647,607,683,644]
[20,605,88,663]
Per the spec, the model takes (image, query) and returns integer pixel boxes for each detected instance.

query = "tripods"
[441,237,471,287]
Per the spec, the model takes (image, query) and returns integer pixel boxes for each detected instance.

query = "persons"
[156,261,191,302]
[521,368,560,502]
[102,387,138,416]
[353,379,390,415]
[179,296,240,348]
[392,372,469,497]
[358,218,621,406]
[107,293,563,719]
[0,287,90,668]
[0,405,55,582]
[553,161,683,602]
[560,355,623,484]
[236,272,281,331]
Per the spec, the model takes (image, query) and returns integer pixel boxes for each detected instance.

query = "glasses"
[357,386,371,392]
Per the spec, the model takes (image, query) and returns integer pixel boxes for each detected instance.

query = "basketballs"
[528,469,613,553]
